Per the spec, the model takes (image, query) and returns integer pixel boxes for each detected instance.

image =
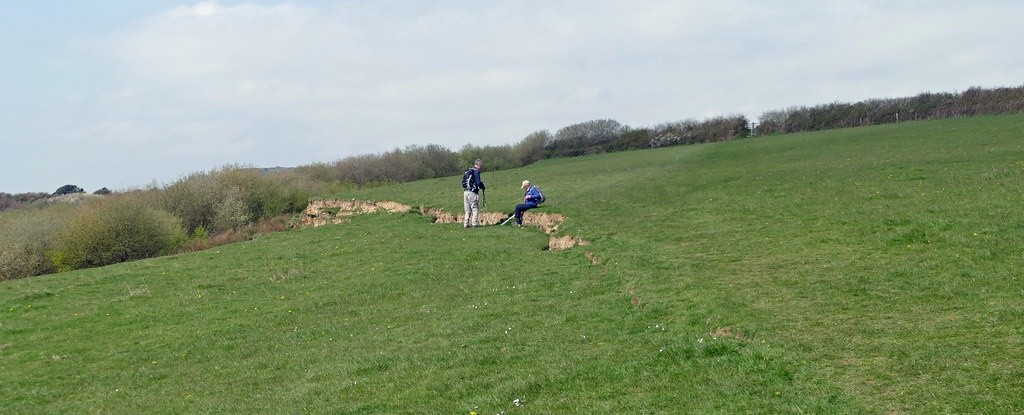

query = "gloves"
[525,195,531,200]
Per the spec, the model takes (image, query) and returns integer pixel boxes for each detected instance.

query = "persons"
[463,159,485,228]
[514,180,540,228]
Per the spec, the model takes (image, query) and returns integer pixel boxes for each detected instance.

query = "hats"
[521,180,530,188]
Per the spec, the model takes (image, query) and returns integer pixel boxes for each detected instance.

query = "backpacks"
[462,168,478,190]
[531,186,546,204]
